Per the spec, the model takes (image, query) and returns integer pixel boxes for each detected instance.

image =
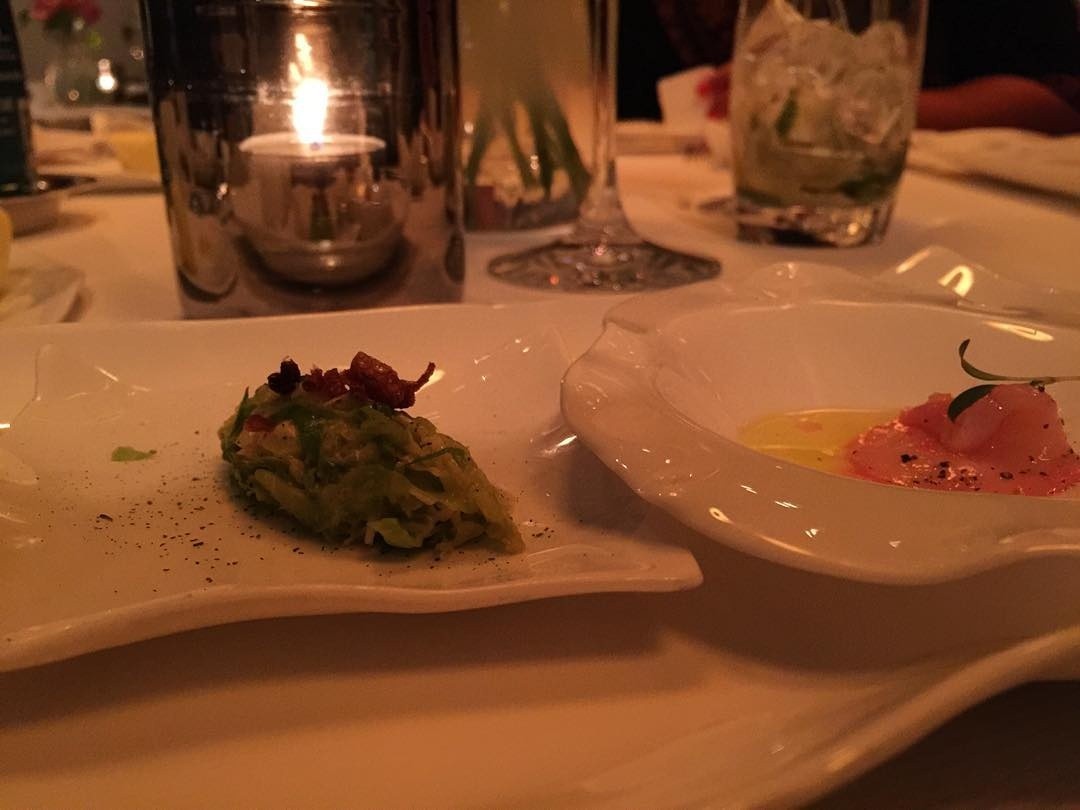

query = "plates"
[0,77,1080,668]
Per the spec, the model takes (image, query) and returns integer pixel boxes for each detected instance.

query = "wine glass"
[481,2,723,292]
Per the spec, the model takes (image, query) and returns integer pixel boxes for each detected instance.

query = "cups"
[141,0,469,320]
[714,2,933,250]
[236,131,398,286]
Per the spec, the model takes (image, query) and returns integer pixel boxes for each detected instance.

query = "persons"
[694,0,1079,140]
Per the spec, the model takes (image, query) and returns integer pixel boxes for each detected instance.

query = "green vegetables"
[946,338,1080,424]
